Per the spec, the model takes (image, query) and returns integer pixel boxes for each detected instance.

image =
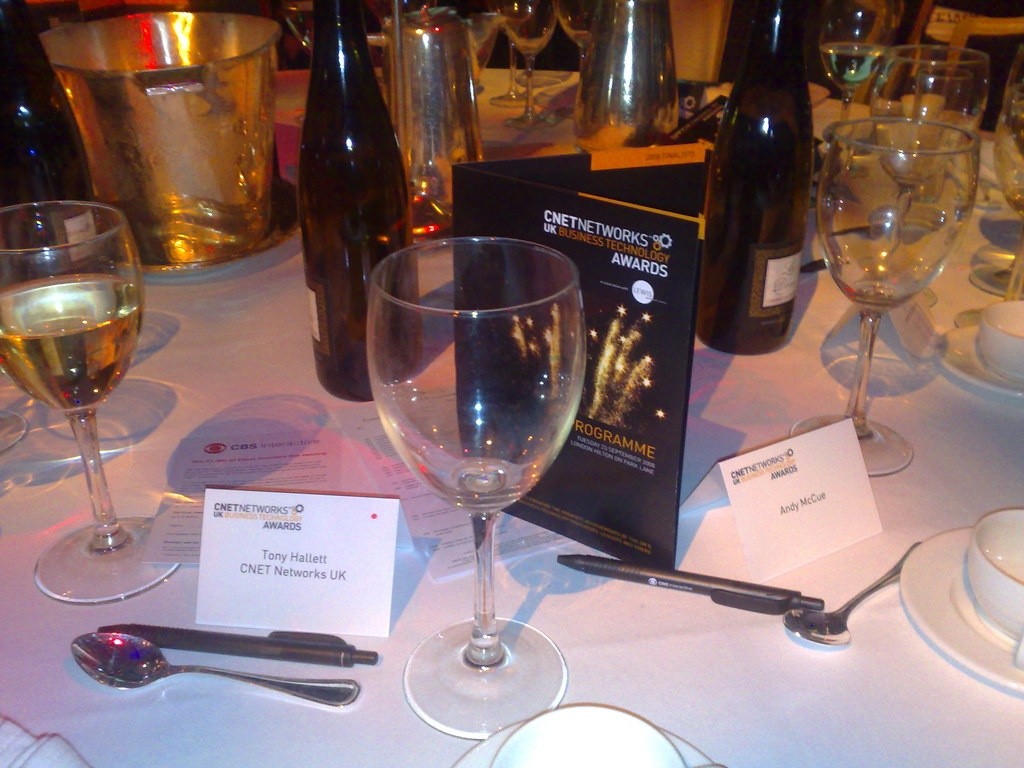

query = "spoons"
[71,633,361,709]
[783,542,921,646]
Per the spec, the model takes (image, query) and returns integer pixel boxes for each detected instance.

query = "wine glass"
[491,0,559,131]
[788,117,983,478]
[489,35,528,109]
[869,43,1023,327]
[0,409,28,453]
[0,200,183,608]
[815,0,907,121]
[367,236,590,744]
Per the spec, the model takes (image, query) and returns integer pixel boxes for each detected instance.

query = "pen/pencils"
[556,553,826,616]
[97,623,379,668]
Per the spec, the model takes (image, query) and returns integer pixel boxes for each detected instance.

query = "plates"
[449,719,725,768]
[932,322,1024,399]
[899,524,1024,697]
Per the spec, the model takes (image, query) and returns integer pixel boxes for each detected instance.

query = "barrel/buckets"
[39,9,282,267]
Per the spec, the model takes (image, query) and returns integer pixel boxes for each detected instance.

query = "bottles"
[696,0,814,358]
[297,0,428,404]
[0,0,93,206]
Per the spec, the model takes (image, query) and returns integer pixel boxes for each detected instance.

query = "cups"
[965,507,1024,669]
[488,702,689,768]
[978,299,1024,385]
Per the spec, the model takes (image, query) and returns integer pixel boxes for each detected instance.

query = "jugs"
[366,6,509,232]
[553,1,681,151]
